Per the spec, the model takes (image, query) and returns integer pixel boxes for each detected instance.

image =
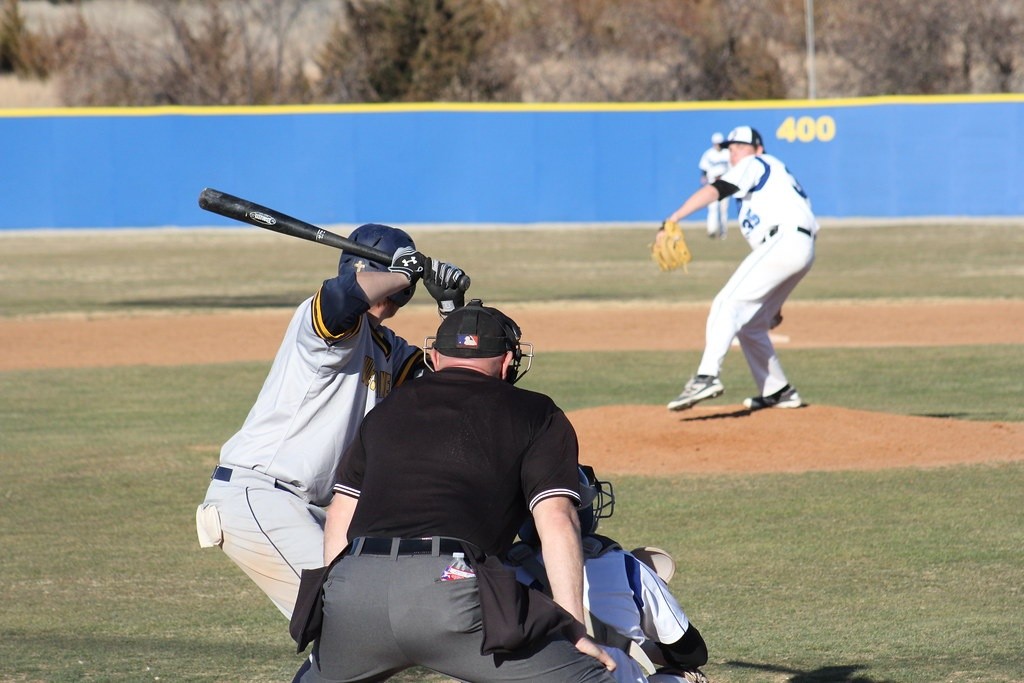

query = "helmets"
[337,223,416,307]
[518,463,594,543]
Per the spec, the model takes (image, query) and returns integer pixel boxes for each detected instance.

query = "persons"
[653,126,819,411]
[196,225,466,623]
[699,133,732,237]
[290,298,619,682]
[509,464,709,683]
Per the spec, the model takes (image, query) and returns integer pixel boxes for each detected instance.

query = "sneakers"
[667,376,724,410]
[744,386,801,409]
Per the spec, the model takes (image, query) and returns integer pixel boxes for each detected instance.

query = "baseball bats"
[197,186,472,292]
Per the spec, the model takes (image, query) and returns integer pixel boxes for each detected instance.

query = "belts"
[211,467,299,496]
[761,227,811,242]
[348,538,464,556]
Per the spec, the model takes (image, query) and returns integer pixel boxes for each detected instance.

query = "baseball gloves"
[647,219,692,277]
[630,546,676,585]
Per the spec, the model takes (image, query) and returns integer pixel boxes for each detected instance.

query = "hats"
[711,133,724,144]
[433,300,515,357]
[720,126,762,148]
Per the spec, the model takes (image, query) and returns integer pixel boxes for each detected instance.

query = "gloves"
[423,256,465,314]
[387,246,426,287]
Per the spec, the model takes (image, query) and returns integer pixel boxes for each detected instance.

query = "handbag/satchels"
[290,542,354,654]
[462,542,575,656]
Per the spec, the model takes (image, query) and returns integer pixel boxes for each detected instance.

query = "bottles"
[452,553,472,571]
